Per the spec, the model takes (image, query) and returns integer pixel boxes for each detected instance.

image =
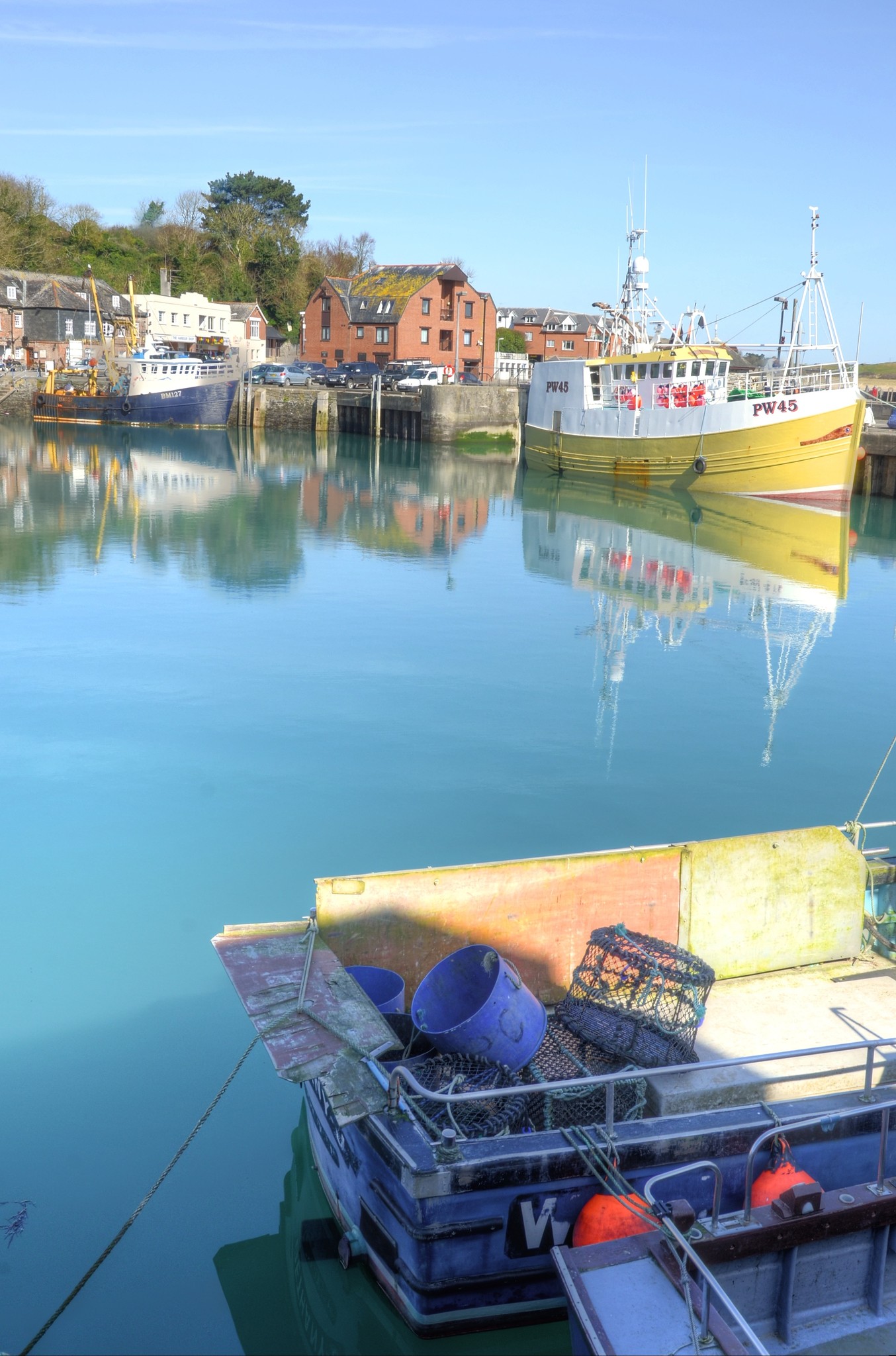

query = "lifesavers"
[122,432,130,444]
[443,365,454,377]
[614,387,627,404]
[37,429,44,441]
[122,402,131,412]
[36,394,46,404]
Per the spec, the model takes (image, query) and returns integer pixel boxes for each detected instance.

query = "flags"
[220,337,223,343]
[685,321,691,343]
[198,337,204,341]
[679,325,683,341]
[205,338,209,343]
[675,325,676,333]
[669,332,675,344]
[698,315,704,329]
[218,337,220,342]
[211,336,216,342]
[215,337,218,342]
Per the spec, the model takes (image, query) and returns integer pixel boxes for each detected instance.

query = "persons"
[865,385,869,399]
[0,355,5,371]
[296,357,299,362]
[873,385,877,389]
[872,387,877,401]
[294,357,296,362]
[6,355,15,372]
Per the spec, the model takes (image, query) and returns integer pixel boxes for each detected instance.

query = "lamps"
[478,340,483,346]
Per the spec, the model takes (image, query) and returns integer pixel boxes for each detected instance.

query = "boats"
[29,265,254,439]
[509,470,857,762]
[520,159,871,518]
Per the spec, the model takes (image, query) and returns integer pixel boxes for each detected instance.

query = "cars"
[264,365,311,387]
[241,364,278,385]
[458,372,482,385]
[0,361,25,370]
[64,358,110,377]
[150,350,234,374]
[311,367,337,386]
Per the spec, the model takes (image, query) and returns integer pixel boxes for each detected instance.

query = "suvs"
[372,356,432,392]
[397,365,455,394]
[292,362,326,379]
[327,361,380,389]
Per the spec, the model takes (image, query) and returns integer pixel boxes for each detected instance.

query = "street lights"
[480,296,488,380]
[454,291,468,385]
[497,338,504,369]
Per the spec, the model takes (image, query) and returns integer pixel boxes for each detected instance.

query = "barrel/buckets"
[411,944,548,1073]
[379,1012,436,1074]
[344,966,404,1014]
